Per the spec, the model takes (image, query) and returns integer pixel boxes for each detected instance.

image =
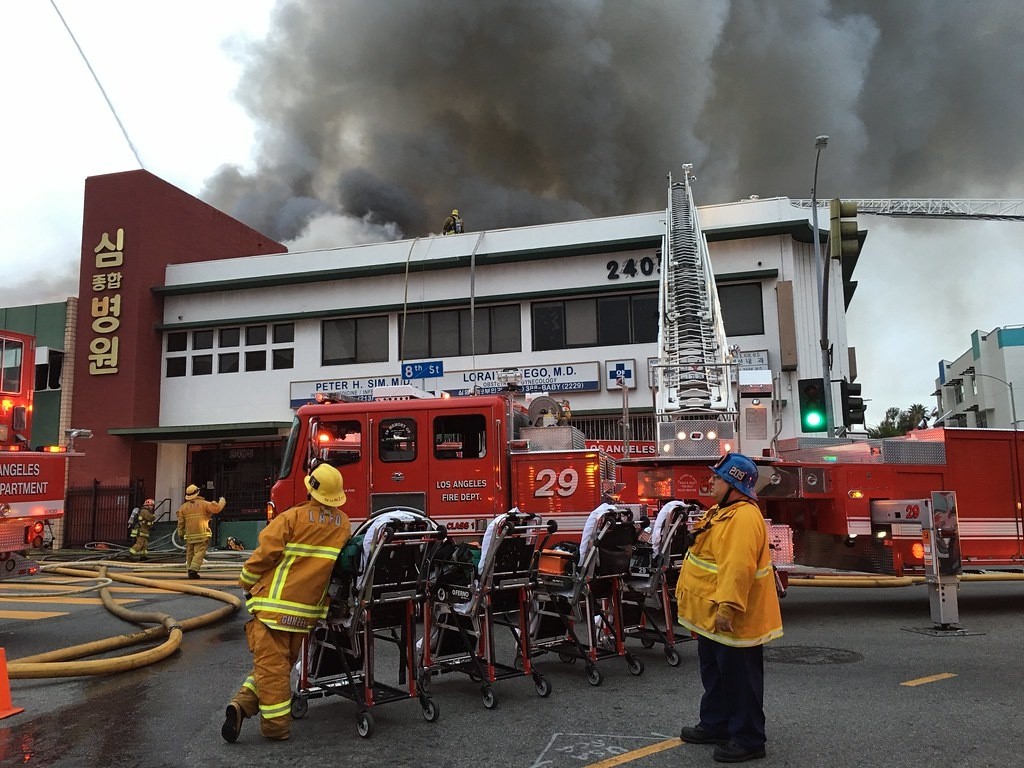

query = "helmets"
[185,484,200,501]
[304,461,346,507]
[707,451,760,501]
[143,499,155,505]
[451,209,459,215]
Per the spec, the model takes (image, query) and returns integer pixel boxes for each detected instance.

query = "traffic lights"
[797,378,826,432]
[841,380,863,429]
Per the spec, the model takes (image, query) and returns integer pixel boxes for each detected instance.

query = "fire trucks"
[262,160,1024,587]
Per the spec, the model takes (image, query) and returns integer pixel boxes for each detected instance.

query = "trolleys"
[622,497,697,665]
[288,521,438,732]
[532,504,644,683]
[435,510,551,707]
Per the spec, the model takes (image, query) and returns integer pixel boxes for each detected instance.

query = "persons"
[177,484,226,580]
[670,452,784,762]
[220,463,350,744]
[443,209,464,235]
[126,499,156,563]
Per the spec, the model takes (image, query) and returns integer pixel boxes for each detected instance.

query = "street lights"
[810,134,845,438]
[962,369,1018,432]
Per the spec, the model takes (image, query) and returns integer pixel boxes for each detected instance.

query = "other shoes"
[124,554,137,562]
[273,731,290,740]
[139,556,151,561]
[221,702,243,744]
[187,569,200,579]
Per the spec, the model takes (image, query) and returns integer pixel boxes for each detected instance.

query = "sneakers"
[681,725,730,744]
[713,740,766,763]
